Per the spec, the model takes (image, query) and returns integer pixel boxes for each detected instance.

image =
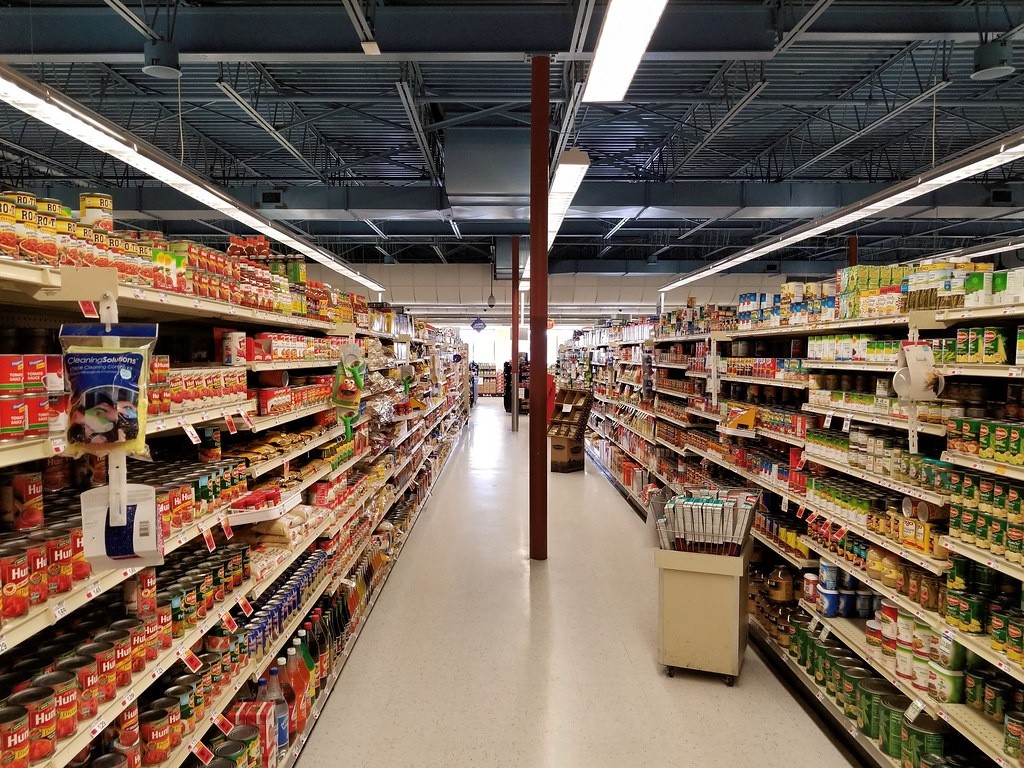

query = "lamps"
[517,148,591,292]
[657,136,1024,293]
[581,1,669,104]
[970,39,1017,81]
[142,38,183,81]
[0,63,388,289]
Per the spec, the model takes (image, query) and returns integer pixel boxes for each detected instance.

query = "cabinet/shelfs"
[0,258,499,768]
[557,300,1023,768]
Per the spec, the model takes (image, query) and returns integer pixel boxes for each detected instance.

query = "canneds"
[649,262,1024,768]
[0,182,410,768]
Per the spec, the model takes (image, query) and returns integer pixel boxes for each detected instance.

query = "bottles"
[232,539,385,764]
